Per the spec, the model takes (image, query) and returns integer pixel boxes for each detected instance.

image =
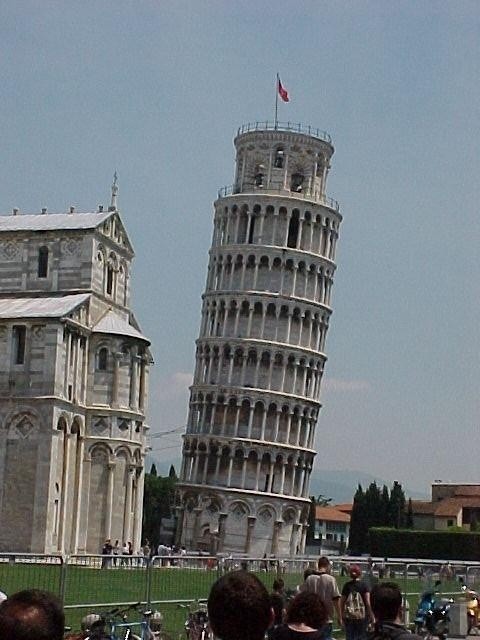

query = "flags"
[278,78,290,102]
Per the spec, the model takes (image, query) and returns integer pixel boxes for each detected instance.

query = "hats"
[350,565,360,577]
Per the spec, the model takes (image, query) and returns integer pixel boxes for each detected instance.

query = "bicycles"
[63,596,213,640]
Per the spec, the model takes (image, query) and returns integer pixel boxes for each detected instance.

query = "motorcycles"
[413,576,480,639]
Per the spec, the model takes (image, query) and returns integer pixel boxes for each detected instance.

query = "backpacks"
[343,591,366,624]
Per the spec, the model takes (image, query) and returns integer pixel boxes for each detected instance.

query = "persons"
[339,564,372,640]
[271,579,288,609]
[0,587,66,639]
[303,556,344,640]
[354,580,423,640]
[273,590,334,640]
[101,537,189,569]
[208,569,271,640]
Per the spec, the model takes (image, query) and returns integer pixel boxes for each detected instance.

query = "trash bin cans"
[448,604,467,639]
